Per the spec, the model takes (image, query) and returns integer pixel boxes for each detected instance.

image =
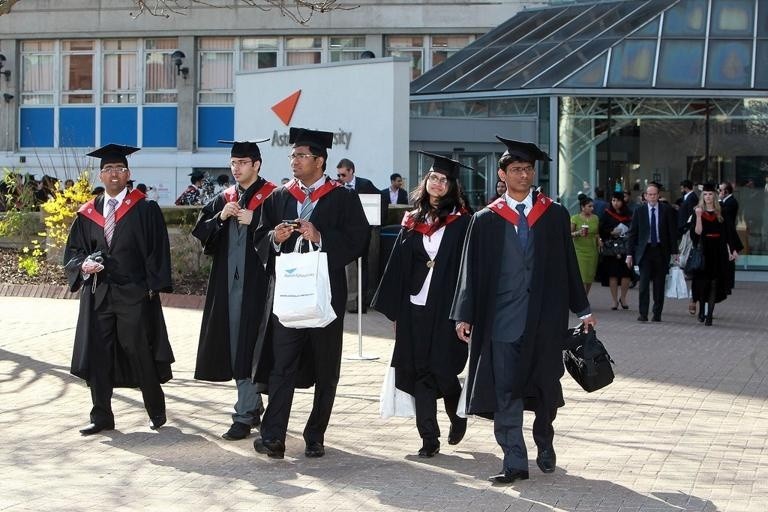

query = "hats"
[290,128,333,150]
[496,136,553,165]
[417,150,474,178]
[703,183,714,191]
[613,192,624,198]
[86,144,140,169]
[218,138,270,158]
[189,171,205,177]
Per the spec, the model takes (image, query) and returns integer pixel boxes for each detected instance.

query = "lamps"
[0,54,7,78]
[171,50,185,76]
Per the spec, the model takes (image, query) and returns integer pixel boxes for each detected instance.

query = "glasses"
[428,175,449,185]
[338,174,345,177]
[100,168,130,172]
[230,161,254,166]
[509,166,534,173]
[287,154,317,159]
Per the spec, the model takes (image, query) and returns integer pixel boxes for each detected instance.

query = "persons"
[1,172,148,211]
[336,159,384,194]
[64,143,175,436]
[570,180,745,327]
[370,149,477,458]
[380,173,408,203]
[176,170,230,204]
[192,139,281,439]
[488,179,507,205]
[449,135,597,483]
[251,128,371,458]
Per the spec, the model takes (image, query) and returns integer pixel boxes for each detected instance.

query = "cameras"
[279,218,300,233]
[89,252,106,265]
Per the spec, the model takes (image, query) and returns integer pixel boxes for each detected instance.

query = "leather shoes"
[488,469,529,483]
[228,422,324,458]
[619,298,628,309]
[419,438,440,457]
[537,443,556,472]
[348,308,366,313]
[150,414,166,430]
[611,302,618,310]
[79,423,114,434]
[448,431,465,444]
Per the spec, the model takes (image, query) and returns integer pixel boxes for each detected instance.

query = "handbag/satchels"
[273,234,337,329]
[664,267,688,299]
[564,323,615,392]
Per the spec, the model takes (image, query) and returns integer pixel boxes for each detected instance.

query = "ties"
[515,204,528,250]
[651,208,657,244]
[104,200,118,247]
[300,188,314,220]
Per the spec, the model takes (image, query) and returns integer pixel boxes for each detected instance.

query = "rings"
[241,208,247,212]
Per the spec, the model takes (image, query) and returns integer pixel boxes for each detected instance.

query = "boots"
[689,301,712,326]
